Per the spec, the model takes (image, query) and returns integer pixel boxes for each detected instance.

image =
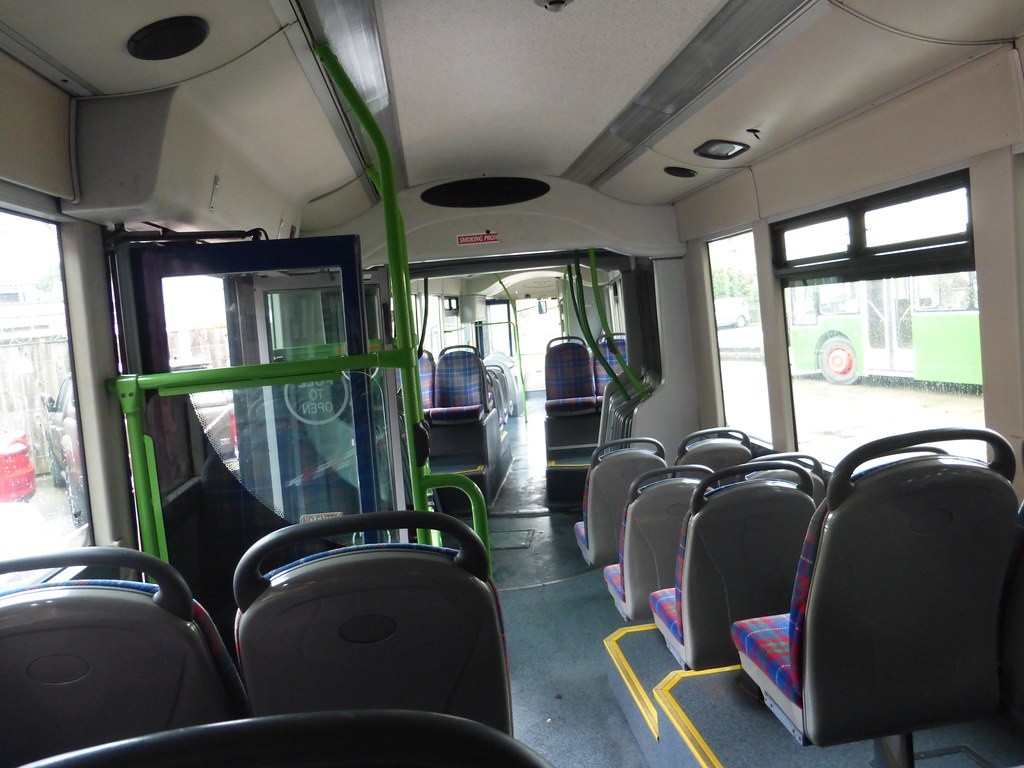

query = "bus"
[760,271,982,387]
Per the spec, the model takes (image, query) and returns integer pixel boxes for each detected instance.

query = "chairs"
[0,332,1024,768]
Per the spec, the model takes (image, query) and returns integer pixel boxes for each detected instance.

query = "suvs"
[715,298,751,328]
[45,368,136,528]
[171,361,240,472]
